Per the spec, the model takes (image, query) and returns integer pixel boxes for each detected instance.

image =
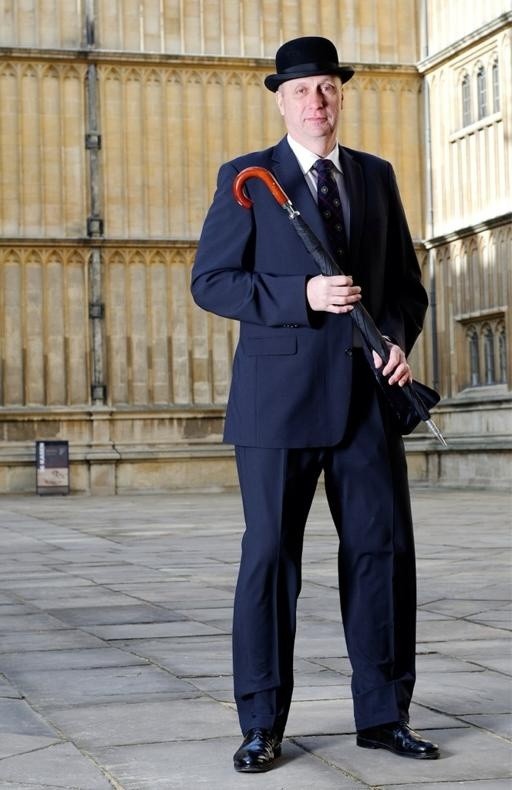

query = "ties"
[311,160,347,275]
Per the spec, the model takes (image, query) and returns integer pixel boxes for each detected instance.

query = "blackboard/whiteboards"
[35,439,69,496]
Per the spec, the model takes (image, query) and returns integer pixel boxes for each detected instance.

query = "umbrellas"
[232,165,448,449]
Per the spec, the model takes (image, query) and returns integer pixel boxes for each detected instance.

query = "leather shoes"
[233,728,282,773]
[357,721,440,760]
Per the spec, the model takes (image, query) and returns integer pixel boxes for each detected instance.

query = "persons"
[189,37,442,773]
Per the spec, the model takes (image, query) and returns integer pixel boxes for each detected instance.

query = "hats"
[265,36,355,93]
